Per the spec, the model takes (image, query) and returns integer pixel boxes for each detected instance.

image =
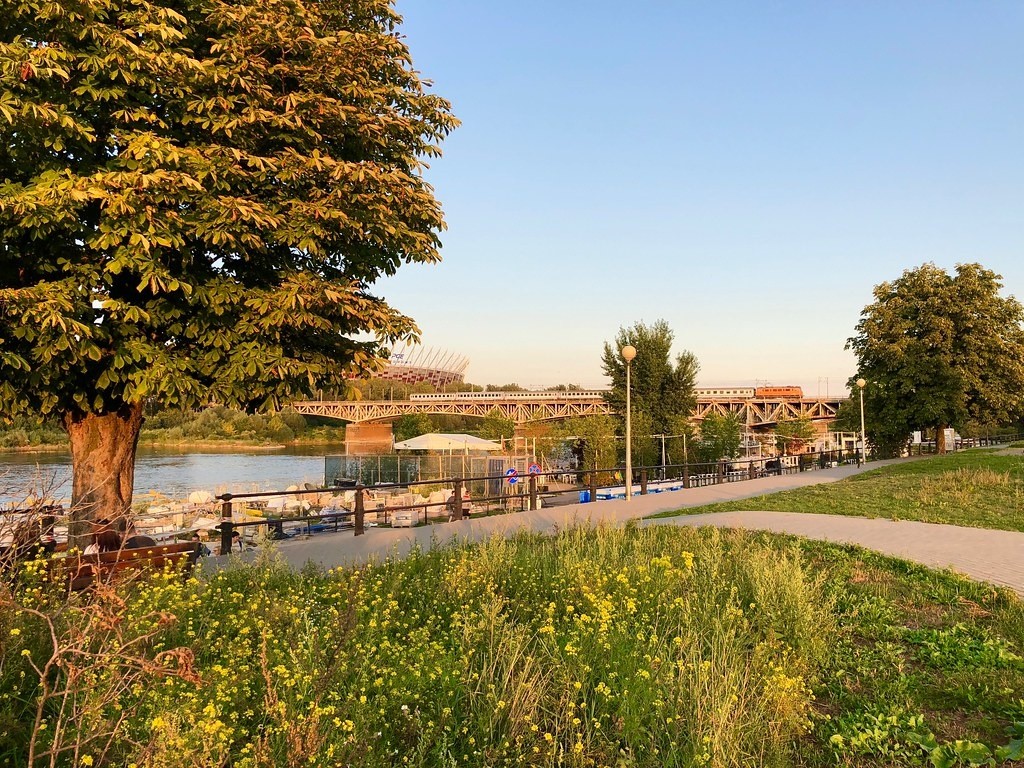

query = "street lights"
[856,378,867,466]
[622,345,638,502]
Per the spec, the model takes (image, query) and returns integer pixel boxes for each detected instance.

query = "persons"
[182,497,307,558]
[10,506,57,560]
[355,472,457,501]
[612,471,623,485]
[445,491,456,523]
[728,451,785,482]
[462,491,472,520]
[83,519,155,565]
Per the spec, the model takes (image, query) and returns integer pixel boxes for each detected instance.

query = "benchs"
[15,541,200,599]
